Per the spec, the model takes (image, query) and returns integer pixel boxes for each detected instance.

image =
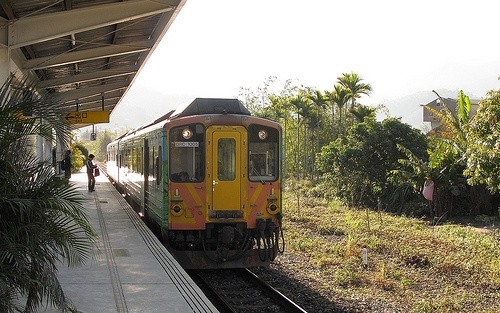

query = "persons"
[65,150,73,183]
[87,154,98,193]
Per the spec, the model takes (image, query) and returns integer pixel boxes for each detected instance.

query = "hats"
[66,150,71,153]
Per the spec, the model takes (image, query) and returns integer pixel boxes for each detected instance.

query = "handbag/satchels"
[95,169,99,176]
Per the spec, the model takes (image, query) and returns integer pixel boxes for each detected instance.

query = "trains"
[106,97,285,268]
